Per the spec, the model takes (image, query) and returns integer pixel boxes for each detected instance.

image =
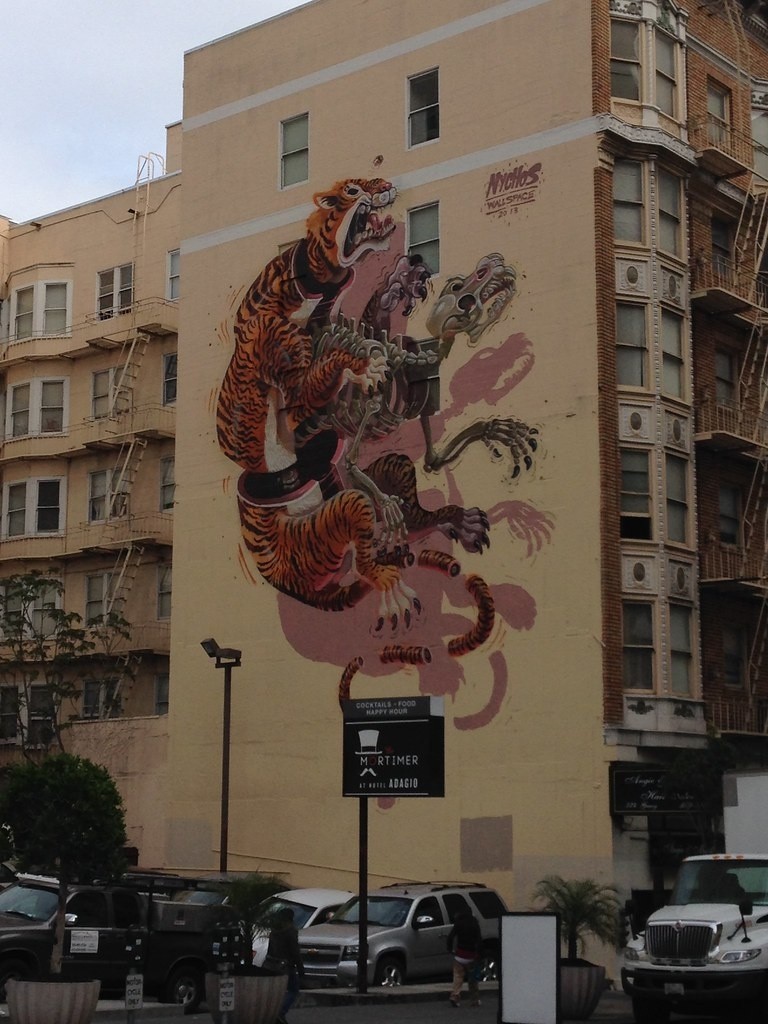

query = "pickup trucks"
[0,870,236,1013]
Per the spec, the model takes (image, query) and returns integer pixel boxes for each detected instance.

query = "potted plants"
[199,867,289,1023]
[526,875,625,1021]
[0,753,139,1024]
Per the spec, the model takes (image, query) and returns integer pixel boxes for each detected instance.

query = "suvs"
[620,854,768,1024]
[296,879,510,988]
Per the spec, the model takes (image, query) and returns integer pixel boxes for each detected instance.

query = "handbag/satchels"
[262,954,289,974]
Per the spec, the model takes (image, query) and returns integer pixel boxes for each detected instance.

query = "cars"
[245,887,355,967]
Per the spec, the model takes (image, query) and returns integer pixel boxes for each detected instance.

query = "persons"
[265,906,305,1024]
[444,904,483,1007]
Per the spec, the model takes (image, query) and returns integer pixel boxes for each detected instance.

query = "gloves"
[297,962,305,977]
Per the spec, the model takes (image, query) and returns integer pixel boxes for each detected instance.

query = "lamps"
[29,222,42,227]
[128,209,135,214]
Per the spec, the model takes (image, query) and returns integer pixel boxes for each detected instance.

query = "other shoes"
[278,1016,288,1024]
[450,997,461,1008]
[473,1001,483,1008]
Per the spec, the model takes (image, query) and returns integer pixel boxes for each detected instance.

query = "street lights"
[198,637,243,880]
